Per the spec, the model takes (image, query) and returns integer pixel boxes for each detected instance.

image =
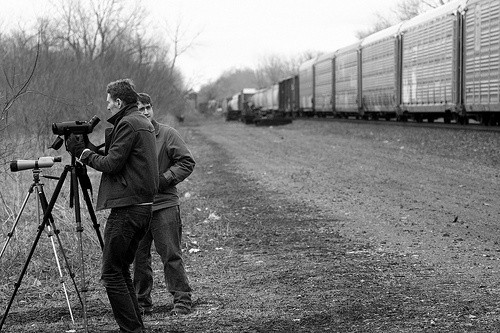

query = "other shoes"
[169,306,192,316]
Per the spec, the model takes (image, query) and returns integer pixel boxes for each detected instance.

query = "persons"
[66,80,159,333]
[133,92,196,316]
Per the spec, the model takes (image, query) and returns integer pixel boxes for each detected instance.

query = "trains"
[222,0,500,125]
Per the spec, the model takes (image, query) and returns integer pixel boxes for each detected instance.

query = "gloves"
[66,133,86,159]
[159,174,169,192]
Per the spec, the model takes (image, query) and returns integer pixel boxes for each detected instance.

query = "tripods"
[0,169,83,325]
[0,135,105,332]
